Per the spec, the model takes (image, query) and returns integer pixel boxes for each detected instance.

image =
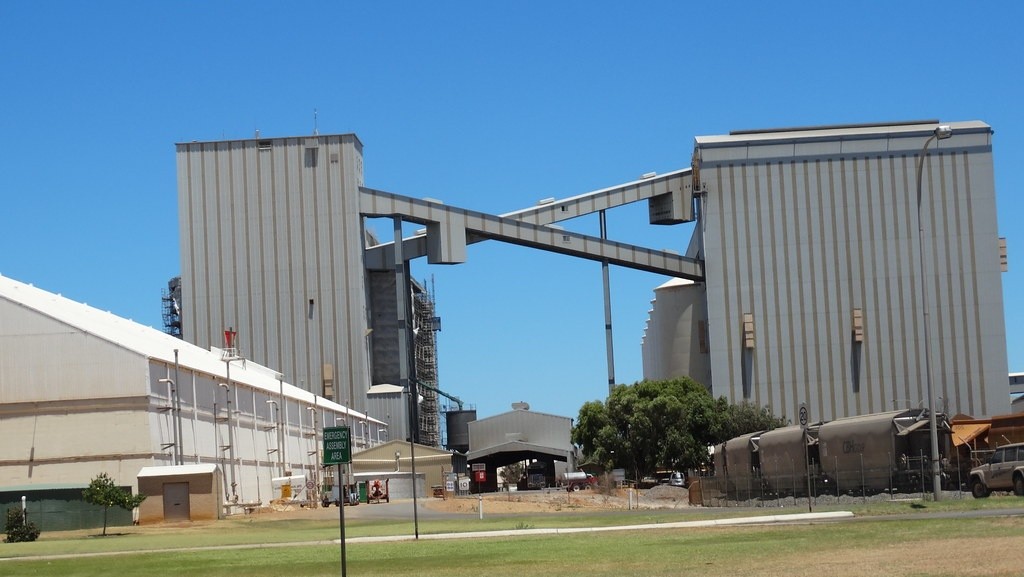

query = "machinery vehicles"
[561,471,601,493]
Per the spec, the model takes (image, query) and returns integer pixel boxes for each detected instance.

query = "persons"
[371,479,384,504]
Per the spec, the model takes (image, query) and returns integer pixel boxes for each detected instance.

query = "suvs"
[969,443,1024,499]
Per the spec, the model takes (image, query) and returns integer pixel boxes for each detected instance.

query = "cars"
[639,477,658,487]
[670,472,685,486]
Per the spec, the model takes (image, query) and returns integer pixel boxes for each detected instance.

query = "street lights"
[380,429,387,439]
[402,376,419,538]
[360,420,368,446]
[157,379,179,466]
[217,382,235,499]
[267,401,281,478]
[306,407,320,489]
[336,417,346,427]
